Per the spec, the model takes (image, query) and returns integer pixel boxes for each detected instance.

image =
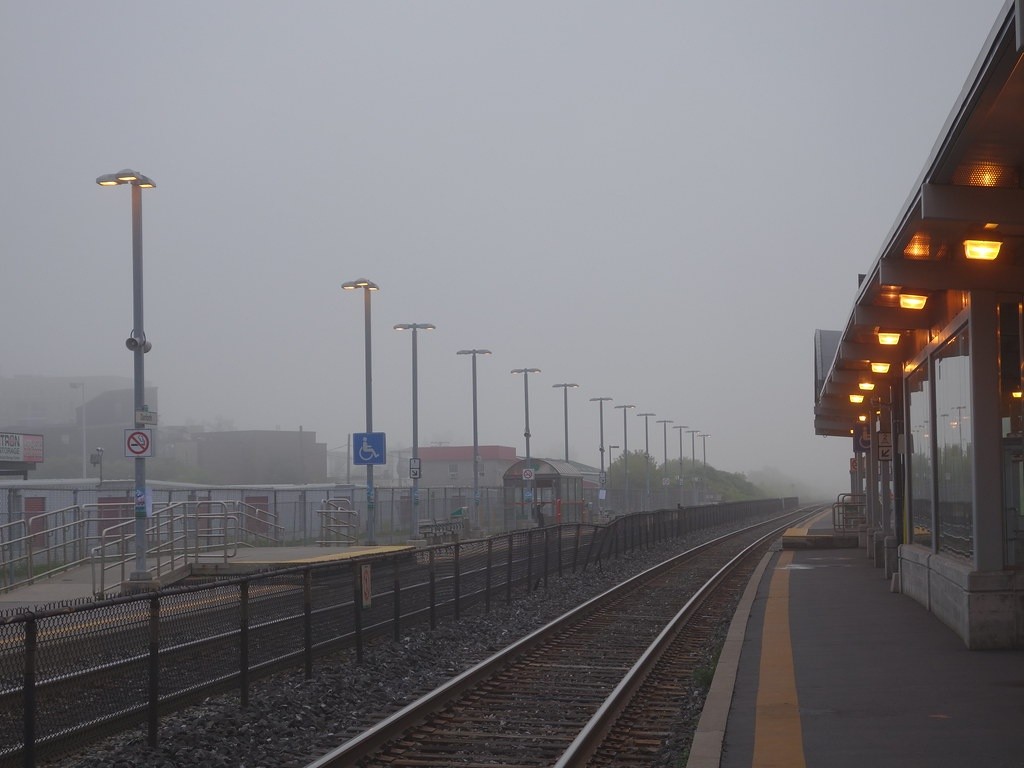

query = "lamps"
[849,394,864,403]
[963,239,1003,261]
[878,333,901,345]
[859,415,866,421]
[871,362,891,373]
[858,383,876,391]
[898,293,928,310]
[1012,391,1023,398]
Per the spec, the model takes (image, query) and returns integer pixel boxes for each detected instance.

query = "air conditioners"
[850,429,854,434]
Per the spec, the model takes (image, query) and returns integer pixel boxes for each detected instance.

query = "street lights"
[95,167,159,592]
[614,404,637,513]
[684,430,700,481]
[551,382,579,466]
[342,278,378,545]
[392,322,436,547]
[510,367,541,526]
[636,412,655,494]
[456,348,491,530]
[672,425,688,505]
[590,397,614,472]
[655,420,673,507]
[696,433,712,475]
